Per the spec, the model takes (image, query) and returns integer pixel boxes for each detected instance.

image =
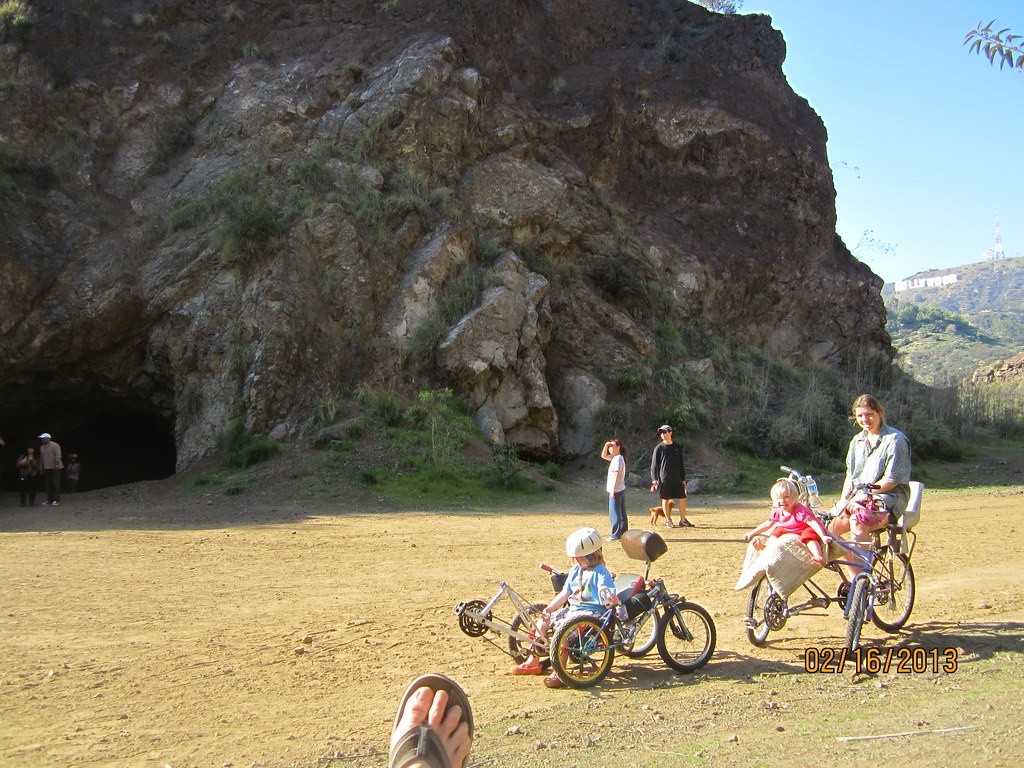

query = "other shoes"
[605,536,614,542]
[679,518,695,527]
[667,519,674,527]
[42,500,61,506]
[510,661,542,675]
[543,671,565,688]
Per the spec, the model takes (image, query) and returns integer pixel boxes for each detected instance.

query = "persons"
[67,454,80,493]
[16,445,38,507]
[601,439,628,543]
[650,424,695,529]
[387,682,472,768]
[38,433,64,505]
[744,476,834,563]
[827,393,912,582]
[509,526,620,689]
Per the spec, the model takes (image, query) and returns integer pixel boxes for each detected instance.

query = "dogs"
[649,499,676,526]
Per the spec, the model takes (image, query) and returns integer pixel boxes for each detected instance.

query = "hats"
[71,454,78,458]
[657,425,672,434]
[39,433,51,438]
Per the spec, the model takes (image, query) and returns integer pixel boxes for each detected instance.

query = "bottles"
[806,475,821,508]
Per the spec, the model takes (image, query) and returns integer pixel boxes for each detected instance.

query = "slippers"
[388,672,474,768]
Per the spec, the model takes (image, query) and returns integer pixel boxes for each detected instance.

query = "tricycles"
[509,528,717,689]
[744,466,924,661]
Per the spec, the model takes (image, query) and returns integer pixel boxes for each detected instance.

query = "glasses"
[659,430,666,434]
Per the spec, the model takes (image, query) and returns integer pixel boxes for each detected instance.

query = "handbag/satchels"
[20,470,32,484]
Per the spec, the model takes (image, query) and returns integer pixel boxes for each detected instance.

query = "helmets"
[854,500,889,528]
[566,527,602,558]
